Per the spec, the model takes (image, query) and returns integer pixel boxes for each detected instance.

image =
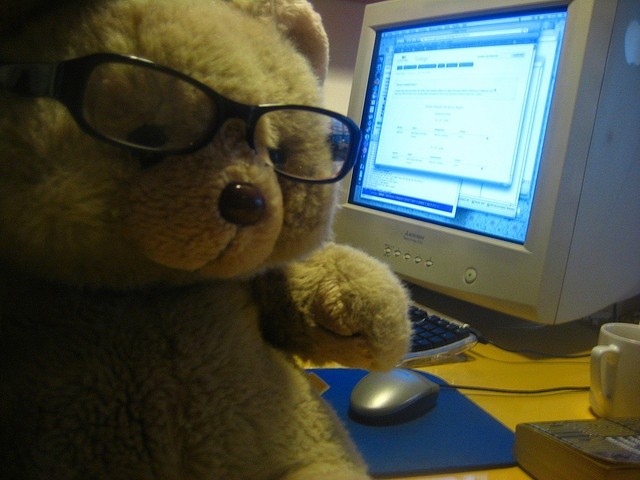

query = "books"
[513,417,640,479]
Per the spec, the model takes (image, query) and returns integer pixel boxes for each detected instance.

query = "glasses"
[12,51,362,184]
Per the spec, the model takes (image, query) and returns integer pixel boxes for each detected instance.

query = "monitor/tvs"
[331,1,640,329]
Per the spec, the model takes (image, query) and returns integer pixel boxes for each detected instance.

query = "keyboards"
[396,306,479,368]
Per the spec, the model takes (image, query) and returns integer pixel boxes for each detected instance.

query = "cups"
[588,321,640,422]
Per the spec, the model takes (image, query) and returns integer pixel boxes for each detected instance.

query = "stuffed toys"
[0,1,411,479]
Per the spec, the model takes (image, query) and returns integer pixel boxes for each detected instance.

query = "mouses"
[349,367,441,426]
